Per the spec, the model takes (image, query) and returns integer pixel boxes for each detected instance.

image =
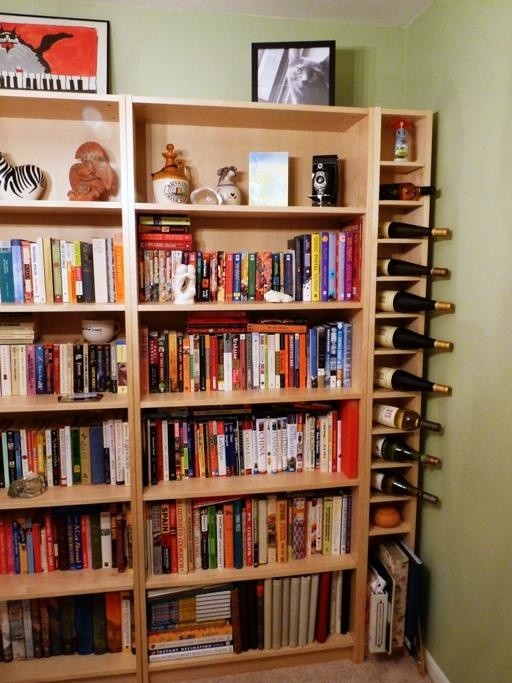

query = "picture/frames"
[247,37,337,106]
[0,11,110,95]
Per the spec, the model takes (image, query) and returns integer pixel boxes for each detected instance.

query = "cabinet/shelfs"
[357,108,437,663]
[0,90,144,682]
[126,94,377,674]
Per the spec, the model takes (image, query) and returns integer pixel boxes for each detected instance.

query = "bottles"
[371,180,457,505]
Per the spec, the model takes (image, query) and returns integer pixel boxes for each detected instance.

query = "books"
[139,216,361,664]
[368,539,424,655]
[0,231,136,662]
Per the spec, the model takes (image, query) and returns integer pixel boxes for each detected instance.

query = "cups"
[80,316,122,345]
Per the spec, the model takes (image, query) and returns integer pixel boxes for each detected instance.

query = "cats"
[286,48,330,105]
